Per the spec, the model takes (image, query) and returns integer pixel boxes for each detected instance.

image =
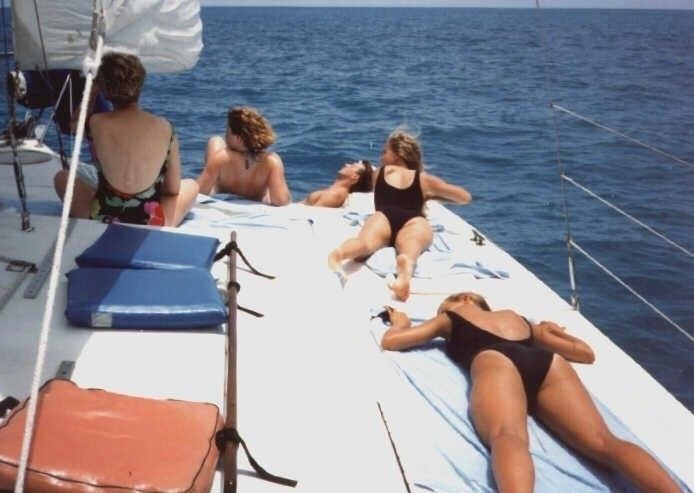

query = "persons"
[382,292,684,493]
[299,160,374,208]
[327,132,472,302]
[54,51,200,228]
[198,107,291,206]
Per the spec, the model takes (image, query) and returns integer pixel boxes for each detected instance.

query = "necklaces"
[230,147,258,170]
